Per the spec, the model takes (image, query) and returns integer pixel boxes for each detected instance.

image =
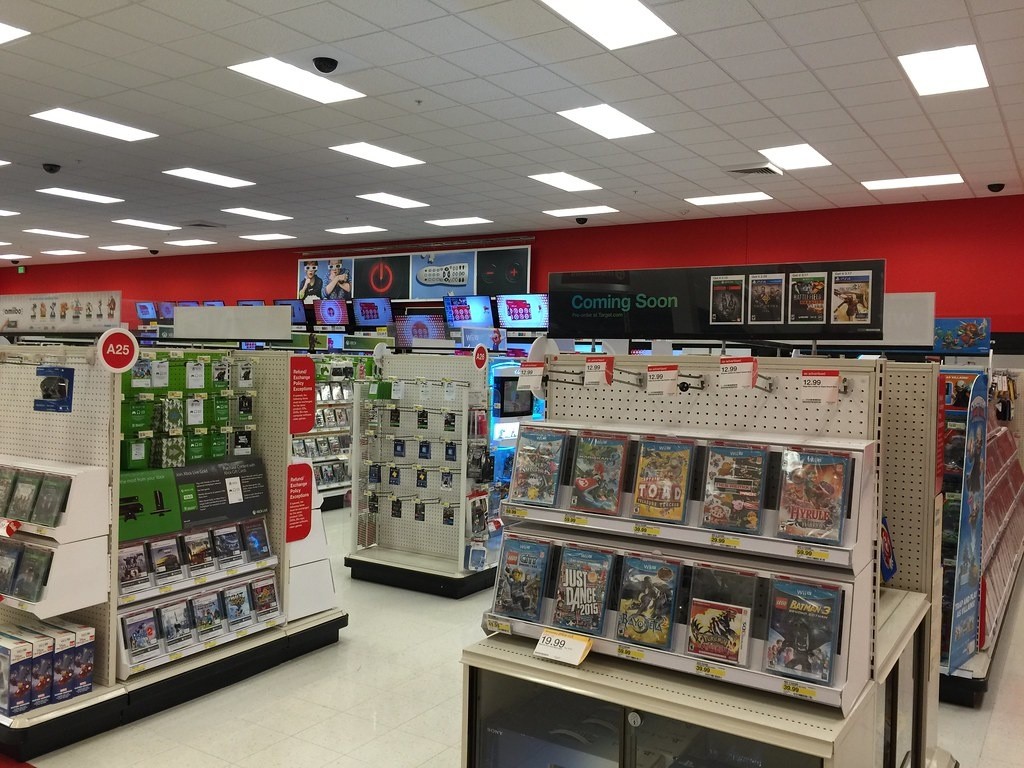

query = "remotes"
[417,263,468,285]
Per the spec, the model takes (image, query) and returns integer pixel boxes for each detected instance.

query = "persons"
[718,294,737,319]
[299,261,322,304]
[834,293,868,321]
[792,281,825,298]
[752,286,781,320]
[320,260,352,300]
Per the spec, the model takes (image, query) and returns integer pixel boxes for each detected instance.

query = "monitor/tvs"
[135,293,684,357]
[493,377,533,418]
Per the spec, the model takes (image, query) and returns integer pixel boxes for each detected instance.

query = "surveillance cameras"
[312,57,338,74]
[150,250,159,254]
[43,164,61,173]
[988,183,1005,192]
[576,218,587,225]
[11,260,19,264]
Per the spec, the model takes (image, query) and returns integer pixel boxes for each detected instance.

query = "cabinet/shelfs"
[0,311,1024,768]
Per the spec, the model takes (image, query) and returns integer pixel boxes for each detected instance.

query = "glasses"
[328,264,342,270]
[304,266,317,270]
